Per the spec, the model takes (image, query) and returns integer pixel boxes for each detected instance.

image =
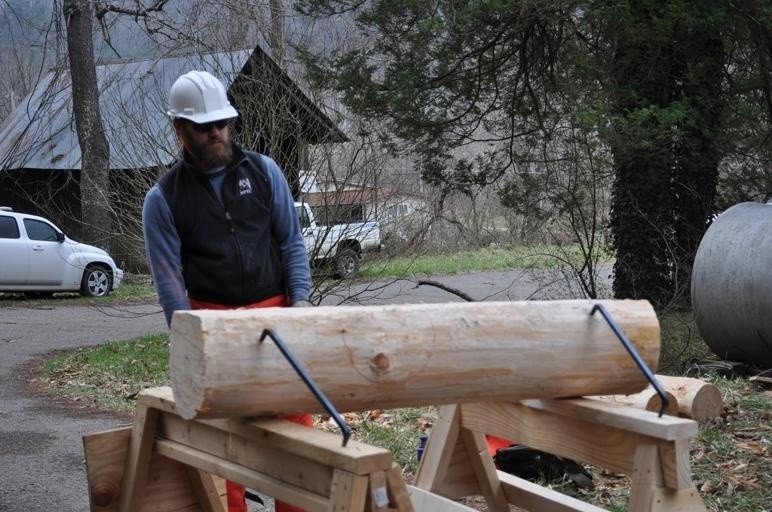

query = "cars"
[0,206,124,298]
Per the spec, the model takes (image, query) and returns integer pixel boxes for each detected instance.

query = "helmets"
[166,69,240,126]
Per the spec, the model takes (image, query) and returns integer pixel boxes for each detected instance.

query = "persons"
[141,67,318,512]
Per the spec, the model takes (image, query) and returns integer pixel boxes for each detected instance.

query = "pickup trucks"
[294,202,385,280]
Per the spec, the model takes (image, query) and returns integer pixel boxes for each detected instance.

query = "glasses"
[174,117,231,135]
